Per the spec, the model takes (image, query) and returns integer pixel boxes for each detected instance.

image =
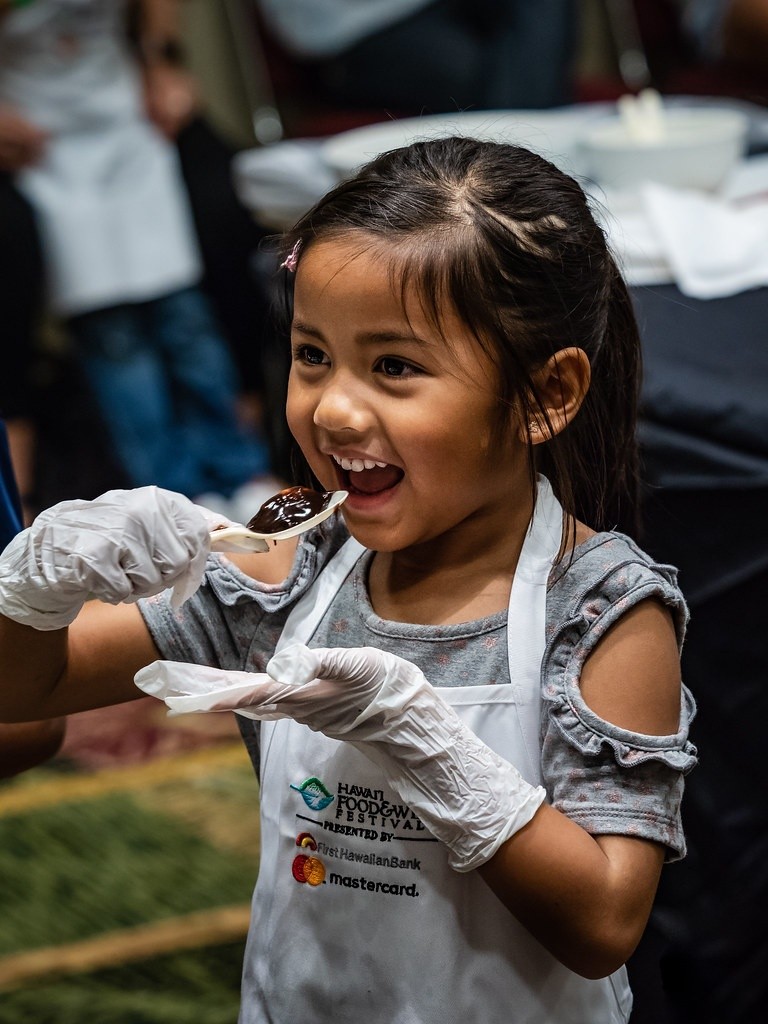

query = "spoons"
[207,488,349,543]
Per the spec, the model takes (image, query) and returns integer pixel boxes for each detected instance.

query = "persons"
[1,0,700,1024]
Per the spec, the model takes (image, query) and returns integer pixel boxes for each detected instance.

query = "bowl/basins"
[234,93,749,230]
